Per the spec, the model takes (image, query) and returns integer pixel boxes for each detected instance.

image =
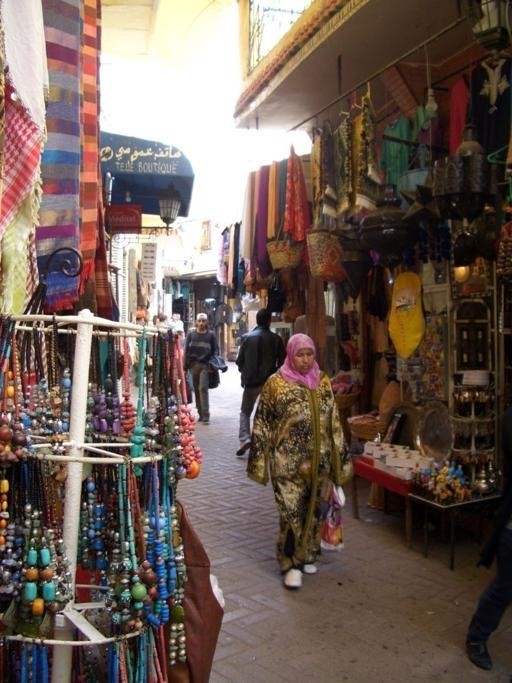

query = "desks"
[408,484,502,571]
[351,456,421,546]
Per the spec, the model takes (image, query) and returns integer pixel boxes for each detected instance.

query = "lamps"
[159,181,181,236]
[472,1,511,62]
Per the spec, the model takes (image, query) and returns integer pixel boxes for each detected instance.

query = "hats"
[196,312,208,321]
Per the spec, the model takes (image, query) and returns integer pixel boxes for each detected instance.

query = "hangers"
[312,115,321,143]
[332,96,350,136]
[347,89,361,112]
[361,78,377,117]
[321,107,332,136]
[488,145,508,163]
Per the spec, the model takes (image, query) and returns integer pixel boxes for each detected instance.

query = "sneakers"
[236,438,252,456]
[283,567,303,588]
[303,563,318,574]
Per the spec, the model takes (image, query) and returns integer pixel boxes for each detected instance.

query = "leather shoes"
[465,638,493,670]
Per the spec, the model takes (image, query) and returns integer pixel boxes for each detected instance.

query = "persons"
[464,403,512,673]
[184,312,220,427]
[135,309,193,406]
[246,330,356,589]
[235,308,286,457]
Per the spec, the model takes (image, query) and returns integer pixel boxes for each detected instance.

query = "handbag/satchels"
[208,369,223,389]
[266,286,283,312]
[264,238,304,269]
[305,229,348,284]
[319,483,345,551]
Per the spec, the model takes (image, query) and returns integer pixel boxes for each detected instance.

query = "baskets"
[348,415,385,439]
[334,390,362,405]
